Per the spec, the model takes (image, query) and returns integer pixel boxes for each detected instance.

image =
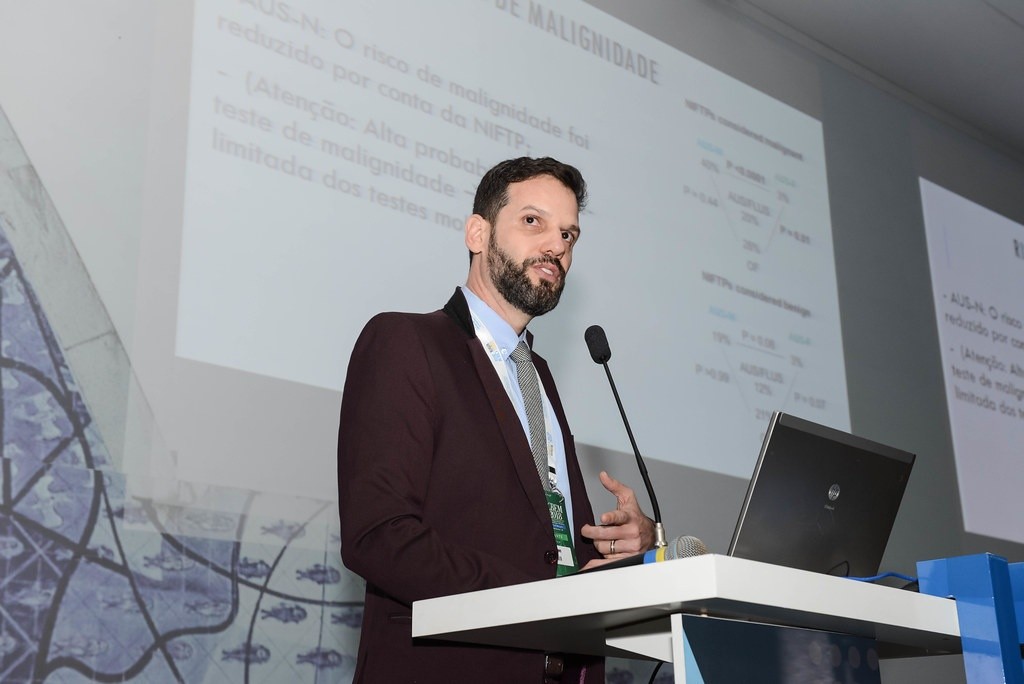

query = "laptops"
[726,410,916,582]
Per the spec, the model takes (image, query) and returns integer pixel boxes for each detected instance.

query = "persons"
[338,156,656,684]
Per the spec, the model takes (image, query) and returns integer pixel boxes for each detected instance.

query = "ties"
[509,342,550,492]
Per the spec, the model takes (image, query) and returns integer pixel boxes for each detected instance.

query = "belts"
[544,655,565,676]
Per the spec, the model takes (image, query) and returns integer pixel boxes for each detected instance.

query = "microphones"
[561,536,709,577]
[585,326,668,549]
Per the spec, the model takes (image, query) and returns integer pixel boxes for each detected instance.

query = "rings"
[610,539,617,554]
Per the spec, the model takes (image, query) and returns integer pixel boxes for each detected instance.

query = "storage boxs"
[915,553,1024,684]
